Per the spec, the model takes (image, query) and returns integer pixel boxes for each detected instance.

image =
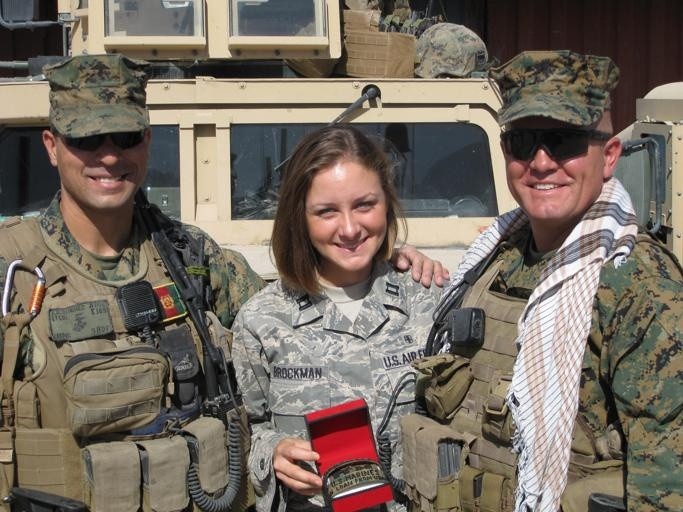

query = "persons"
[0,52,453,511]
[231,124,451,510]
[391,44,683,512]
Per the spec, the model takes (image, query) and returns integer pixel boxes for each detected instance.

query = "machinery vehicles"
[609,82,682,270]
[0,1,524,287]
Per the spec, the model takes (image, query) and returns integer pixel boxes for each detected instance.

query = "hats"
[491,48,622,127]
[41,50,155,139]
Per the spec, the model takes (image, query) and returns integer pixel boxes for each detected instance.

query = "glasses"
[500,127,612,161]
